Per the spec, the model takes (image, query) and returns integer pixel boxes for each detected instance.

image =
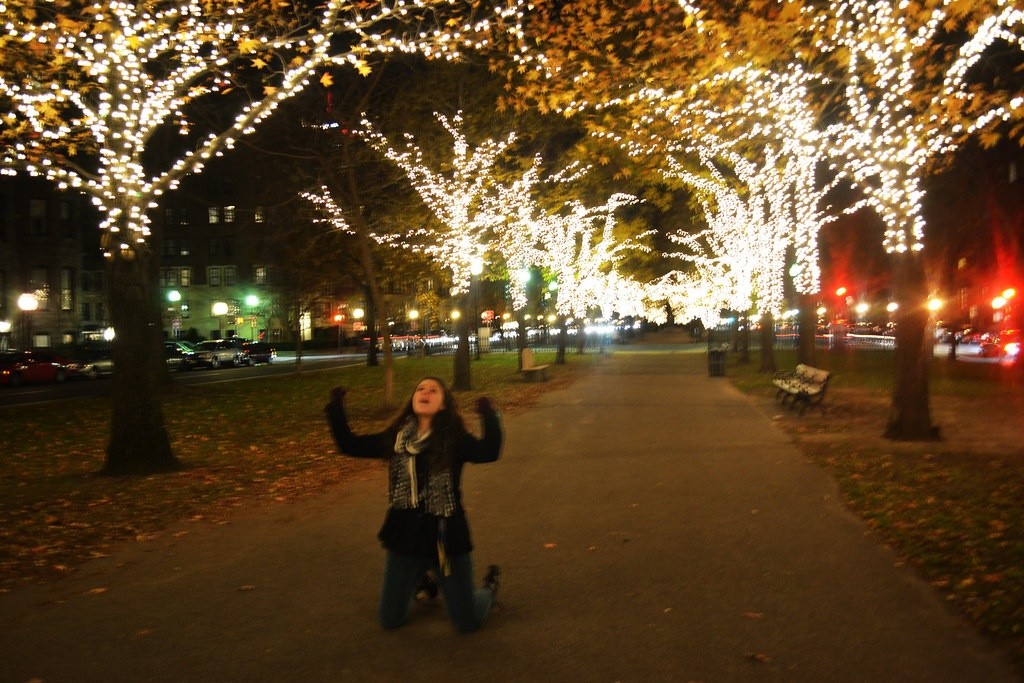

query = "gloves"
[476,397,492,414]
[330,386,346,401]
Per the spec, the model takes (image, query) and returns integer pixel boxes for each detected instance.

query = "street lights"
[17,291,38,350]
[213,302,229,339]
[334,314,345,352]
[246,295,260,341]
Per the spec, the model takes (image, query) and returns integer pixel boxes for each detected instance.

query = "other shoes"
[484,565,499,594]
[418,572,438,598]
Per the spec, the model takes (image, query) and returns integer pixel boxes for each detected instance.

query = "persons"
[322,377,502,634]
[758,312,780,373]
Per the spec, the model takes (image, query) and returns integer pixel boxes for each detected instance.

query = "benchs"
[520,347,550,380]
[771,364,832,418]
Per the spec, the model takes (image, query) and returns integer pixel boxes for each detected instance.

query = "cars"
[0,350,66,387]
[376,334,457,354]
[186,339,244,369]
[72,352,116,380]
[164,341,190,368]
[240,341,275,368]
